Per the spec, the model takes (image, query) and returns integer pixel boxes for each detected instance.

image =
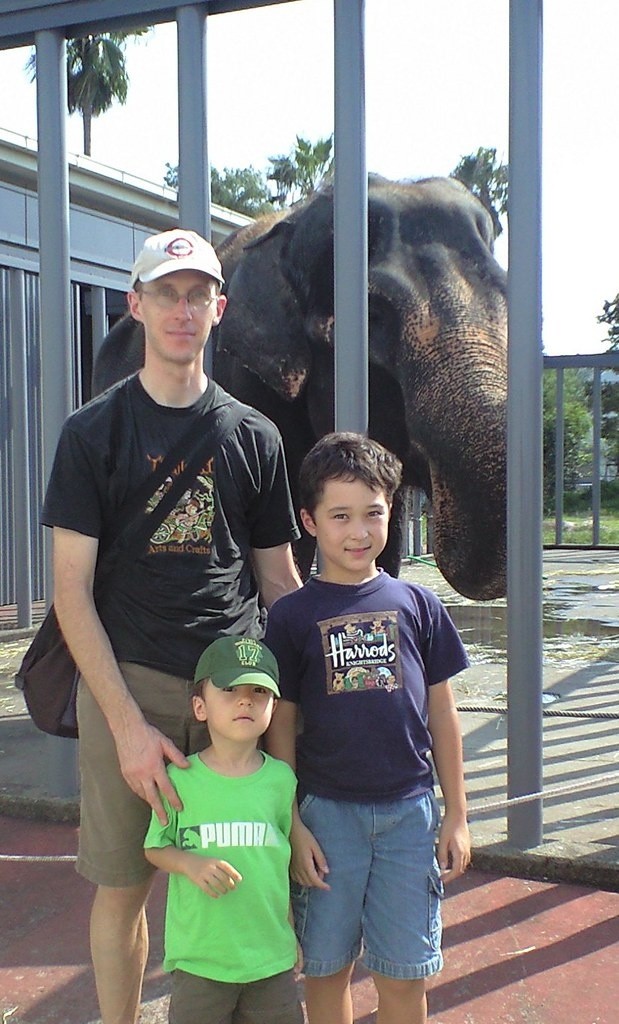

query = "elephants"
[90,173,507,601]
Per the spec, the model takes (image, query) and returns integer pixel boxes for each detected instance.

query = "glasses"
[139,287,219,309]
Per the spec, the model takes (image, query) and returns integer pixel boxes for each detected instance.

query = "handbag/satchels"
[14,604,80,739]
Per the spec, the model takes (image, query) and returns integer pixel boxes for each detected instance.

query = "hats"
[133,229,225,290]
[194,636,280,698]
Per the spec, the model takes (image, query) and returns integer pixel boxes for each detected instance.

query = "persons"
[143,636,303,1024]
[40,229,305,1024]
[265,431,472,1024]
